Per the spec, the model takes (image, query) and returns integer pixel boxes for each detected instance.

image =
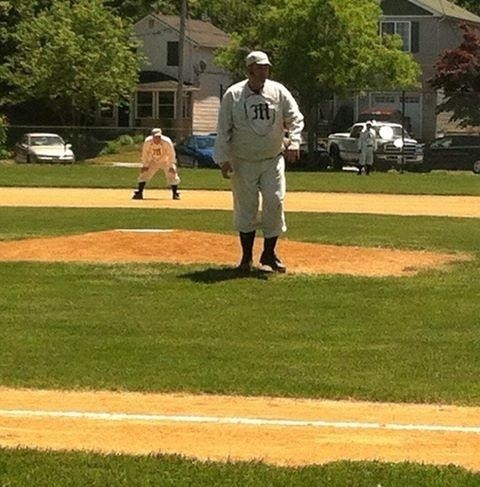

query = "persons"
[211,50,305,275]
[357,121,378,175]
[129,127,181,200]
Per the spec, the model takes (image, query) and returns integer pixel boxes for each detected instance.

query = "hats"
[245,50,273,68]
[150,128,163,139]
[366,120,372,125]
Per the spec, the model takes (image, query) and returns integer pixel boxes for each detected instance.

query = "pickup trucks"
[327,119,424,172]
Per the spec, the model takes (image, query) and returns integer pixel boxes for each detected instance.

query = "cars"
[174,135,222,169]
[13,132,76,164]
[423,135,480,173]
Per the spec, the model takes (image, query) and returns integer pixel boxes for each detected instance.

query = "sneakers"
[173,193,181,200]
[132,192,144,199]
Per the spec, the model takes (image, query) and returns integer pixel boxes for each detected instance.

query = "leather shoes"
[260,248,287,274]
[236,256,254,276]
